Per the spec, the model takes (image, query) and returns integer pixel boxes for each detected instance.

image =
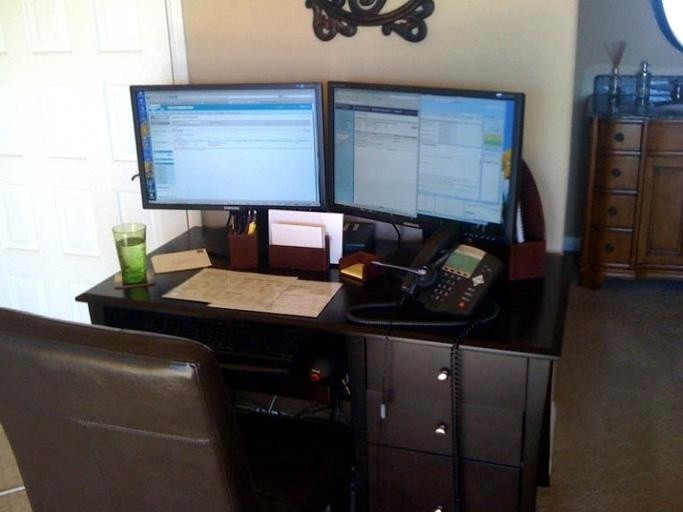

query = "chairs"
[0,304,240,510]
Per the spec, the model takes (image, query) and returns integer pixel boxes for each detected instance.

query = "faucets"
[675,82,682,99]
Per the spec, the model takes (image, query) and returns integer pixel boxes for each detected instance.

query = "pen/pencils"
[229,209,258,235]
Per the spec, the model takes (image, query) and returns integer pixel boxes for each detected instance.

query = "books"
[271,221,325,249]
[268,206,344,266]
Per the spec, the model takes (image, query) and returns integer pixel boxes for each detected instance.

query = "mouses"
[309,357,337,385]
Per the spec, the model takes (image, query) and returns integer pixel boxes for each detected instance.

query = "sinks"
[653,103,682,117]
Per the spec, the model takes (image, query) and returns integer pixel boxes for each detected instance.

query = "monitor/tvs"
[325,80,526,248]
[129,81,328,254]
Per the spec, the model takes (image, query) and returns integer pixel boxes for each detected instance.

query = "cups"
[112,221,147,285]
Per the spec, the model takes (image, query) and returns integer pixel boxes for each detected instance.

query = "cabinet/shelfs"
[577,117,682,294]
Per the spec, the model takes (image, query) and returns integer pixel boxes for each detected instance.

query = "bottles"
[609,67,622,103]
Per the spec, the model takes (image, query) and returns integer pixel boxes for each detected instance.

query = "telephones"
[400,229,506,315]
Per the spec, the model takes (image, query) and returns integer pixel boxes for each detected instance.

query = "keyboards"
[138,315,307,367]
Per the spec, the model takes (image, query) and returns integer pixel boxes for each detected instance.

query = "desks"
[75,225,572,511]
